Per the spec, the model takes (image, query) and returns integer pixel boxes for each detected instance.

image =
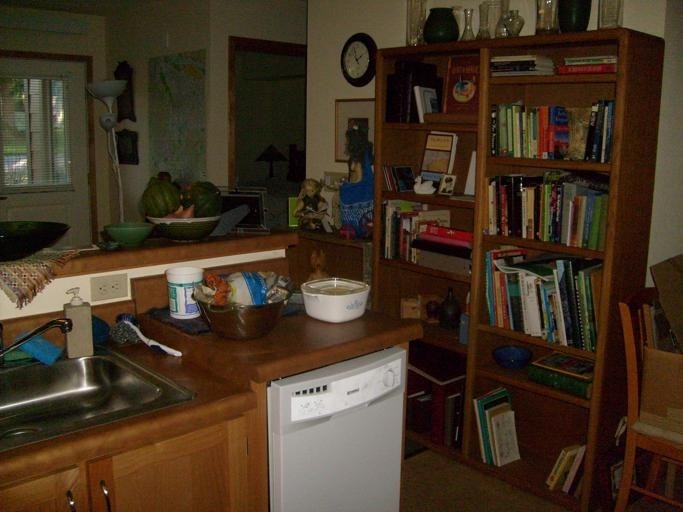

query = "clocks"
[337,26,381,90]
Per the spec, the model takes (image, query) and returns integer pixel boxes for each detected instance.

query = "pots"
[0,221,71,262]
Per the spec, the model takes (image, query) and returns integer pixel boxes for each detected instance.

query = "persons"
[336,141,375,241]
[293,176,330,236]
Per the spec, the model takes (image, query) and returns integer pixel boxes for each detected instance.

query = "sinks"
[1,343,198,450]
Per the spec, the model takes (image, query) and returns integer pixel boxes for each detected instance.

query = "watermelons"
[141,179,180,217]
[181,182,222,217]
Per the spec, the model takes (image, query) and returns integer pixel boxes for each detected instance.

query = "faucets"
[1,318,73,355]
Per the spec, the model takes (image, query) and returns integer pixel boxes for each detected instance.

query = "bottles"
[460,8,475,40]
[440,287,460,329]
[494,0,508,38]
[474,3,491,40]
[504,10,524,38]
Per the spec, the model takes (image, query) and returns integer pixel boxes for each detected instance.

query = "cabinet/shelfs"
[371,23,664,512]
[266,221,370,288]
[0,408,251,511]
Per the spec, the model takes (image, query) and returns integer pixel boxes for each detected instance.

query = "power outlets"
[89,270,136,305]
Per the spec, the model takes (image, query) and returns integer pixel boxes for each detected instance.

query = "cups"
[597,1,620,30]
[536,0,558,35]
[406,7,425,47]
[458,313,468,344]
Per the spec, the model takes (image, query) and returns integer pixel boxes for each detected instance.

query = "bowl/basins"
[189,284,292,341]
[104,223,154,247]
[147,216,219,243]
[299,275,371,322]
[493,344,532,369]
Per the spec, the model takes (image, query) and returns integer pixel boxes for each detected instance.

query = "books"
[486,172,609,253]
[487,248,603,353]
[490,54,619,77]
[527,351,595,399]
[446,394,463,447]
[490,99,615,164]
[381,198,474,275]
[384,52,480,126]
[473,386,520,468]
[421,131,458,188]
[547,444,587,494]
[642,299,681,354]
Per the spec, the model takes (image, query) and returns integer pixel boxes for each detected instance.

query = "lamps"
[86,76,143,226]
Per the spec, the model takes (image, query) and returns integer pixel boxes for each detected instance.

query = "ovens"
[266,345,406,511]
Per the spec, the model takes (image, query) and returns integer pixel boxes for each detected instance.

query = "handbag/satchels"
[339,161,375,238]
[599,417,646,511]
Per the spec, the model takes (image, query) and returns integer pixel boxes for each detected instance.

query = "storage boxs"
[637,341,681,438]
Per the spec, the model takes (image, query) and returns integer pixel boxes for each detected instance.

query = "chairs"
[614,277,681,510]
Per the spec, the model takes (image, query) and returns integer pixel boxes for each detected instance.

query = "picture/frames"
[331,95,377,164]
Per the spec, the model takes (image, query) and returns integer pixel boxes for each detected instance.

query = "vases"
[403,0,622,51]
